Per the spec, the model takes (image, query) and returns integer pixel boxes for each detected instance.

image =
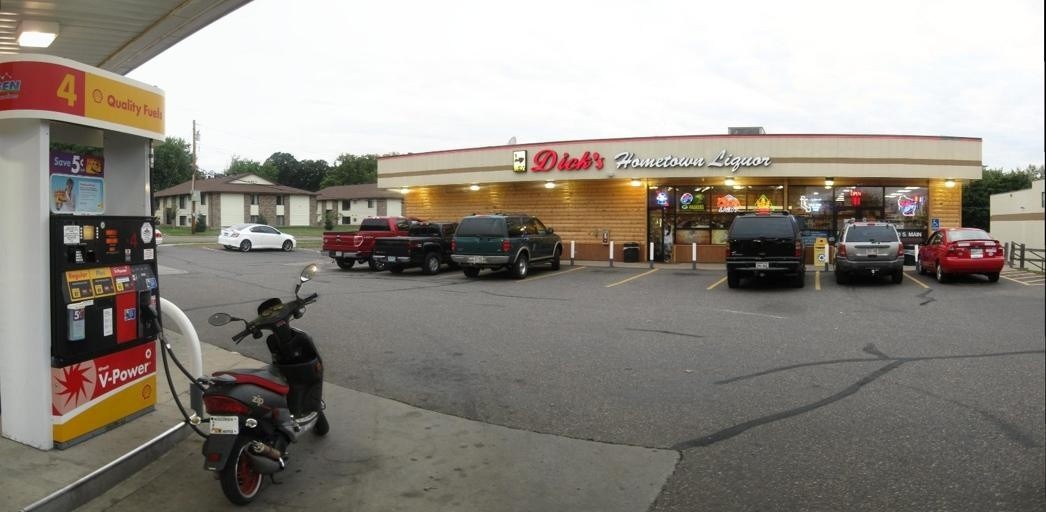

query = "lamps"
[17,20,61,49]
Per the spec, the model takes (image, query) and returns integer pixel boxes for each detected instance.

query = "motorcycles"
[202,264,330,505]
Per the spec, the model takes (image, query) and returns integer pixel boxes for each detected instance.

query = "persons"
[55,177,76,212]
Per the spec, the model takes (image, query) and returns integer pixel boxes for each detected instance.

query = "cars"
[918,227,1005,283]
[218,224,296,252]
[155,229,162,245]
[832,222,905,284]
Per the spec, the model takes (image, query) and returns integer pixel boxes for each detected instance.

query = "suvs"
[450,216,562,279]
[725,212,805,289]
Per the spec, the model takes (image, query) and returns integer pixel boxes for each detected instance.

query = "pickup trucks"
[321,216,460,275]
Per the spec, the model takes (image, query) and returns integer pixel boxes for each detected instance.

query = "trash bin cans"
[904,244,916,266]
[623,242,640,263]
